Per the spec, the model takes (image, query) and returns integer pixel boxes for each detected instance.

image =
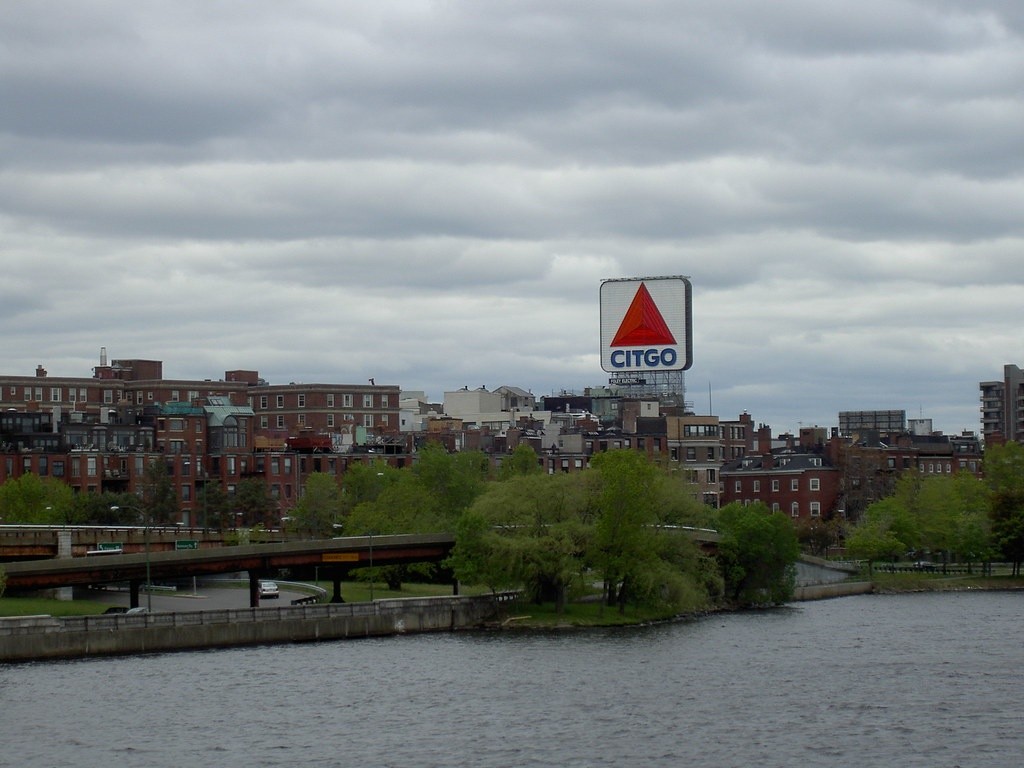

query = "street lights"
[110,506,151,612]
[333,524,374,600]
[185,462,207,527]
[46,507,66,525]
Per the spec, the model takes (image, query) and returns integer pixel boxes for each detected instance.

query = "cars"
[101,607,128,614]
[258,582,279,599]
[126,607,156,613]
